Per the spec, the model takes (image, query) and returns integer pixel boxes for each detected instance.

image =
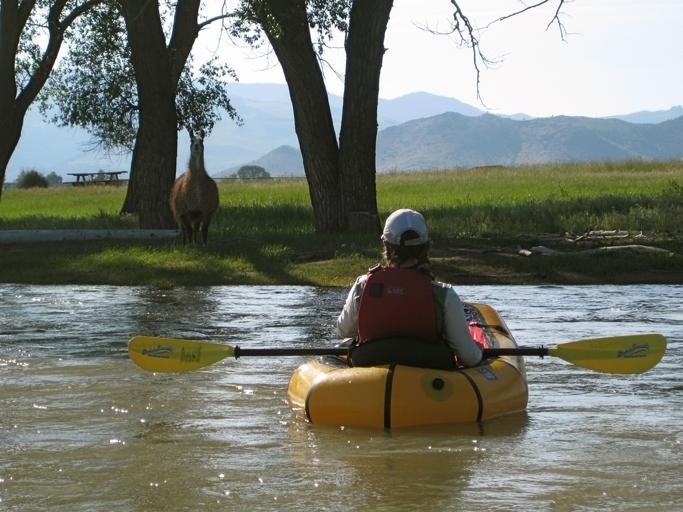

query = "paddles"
[128,335,667,375]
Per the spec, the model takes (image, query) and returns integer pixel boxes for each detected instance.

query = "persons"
[333,206,484,369]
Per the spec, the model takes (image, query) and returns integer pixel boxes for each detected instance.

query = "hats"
[381,209,428,246]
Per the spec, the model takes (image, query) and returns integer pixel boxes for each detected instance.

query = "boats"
[287,301,528,427]
